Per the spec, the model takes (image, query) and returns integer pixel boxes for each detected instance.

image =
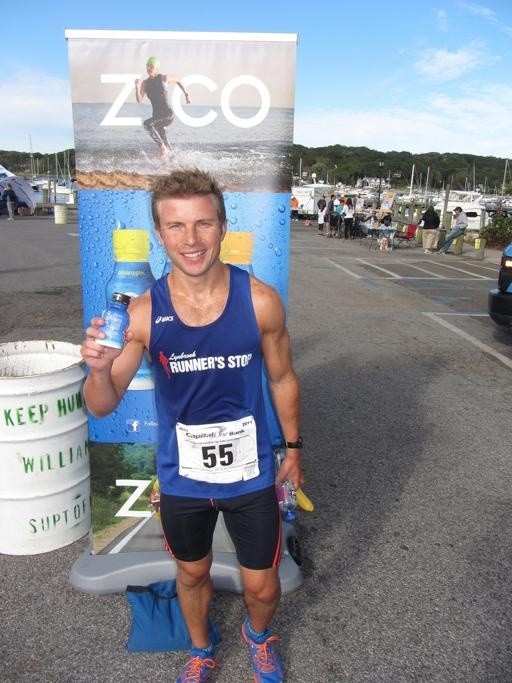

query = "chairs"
[395,223,418,249]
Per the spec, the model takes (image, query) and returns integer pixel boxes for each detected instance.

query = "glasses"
[146,63,155,69]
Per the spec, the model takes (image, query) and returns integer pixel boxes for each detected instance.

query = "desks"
[359,222,398,250]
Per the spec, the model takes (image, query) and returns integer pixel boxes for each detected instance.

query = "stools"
[473,237,487,259]
[453,235,463,255]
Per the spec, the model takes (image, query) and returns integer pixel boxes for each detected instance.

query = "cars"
[488,241,511,328]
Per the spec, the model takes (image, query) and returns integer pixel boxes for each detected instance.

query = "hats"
[382,207,392,212]
[322,195,325,197]
[452,206,461,210]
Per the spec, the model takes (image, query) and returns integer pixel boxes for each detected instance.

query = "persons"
[417,205,440,255]
[134,56,191,158]
[2,184,17,221]
[436,206,467,255]
[398,193,404,205]
[80,170,304,682]
[70,177,78,200]
[290,194,392,239]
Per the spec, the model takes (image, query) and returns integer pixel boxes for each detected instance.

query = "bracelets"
[283,438,303,449]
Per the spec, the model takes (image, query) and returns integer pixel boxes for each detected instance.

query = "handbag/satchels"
[418,220,425,227]
[126,580,222,652]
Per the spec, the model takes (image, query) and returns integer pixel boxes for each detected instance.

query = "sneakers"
[8,217,15,221]
[174,645,219,682]
[243,619,286,683]
[436,251,445,254]
[424,248,432,254]
[318,233,324,235]
[350,235,353,238]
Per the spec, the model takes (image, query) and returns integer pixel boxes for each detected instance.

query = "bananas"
[295,487,314,514]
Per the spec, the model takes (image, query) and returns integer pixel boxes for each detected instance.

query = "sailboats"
[291,153,511,230]
[21,132,76,195]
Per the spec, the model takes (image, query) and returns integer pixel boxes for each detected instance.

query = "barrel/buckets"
[0,339,93,556]
[54,204,69,225]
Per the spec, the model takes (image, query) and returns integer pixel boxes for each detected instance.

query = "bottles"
[274,448,300,521]
[103,228,157,391]
[219,231,254,277]
[96,291,129,349]
[162,253,173,276]
